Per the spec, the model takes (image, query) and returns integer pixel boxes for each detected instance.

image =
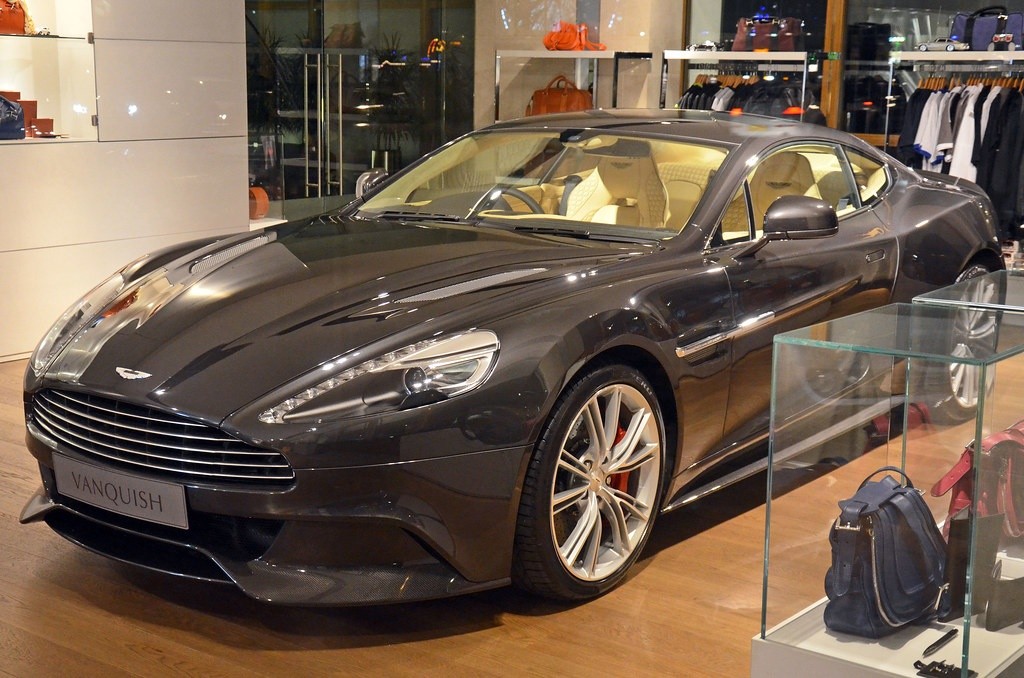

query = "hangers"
[693,63,760,89]
[918,64,1024,93]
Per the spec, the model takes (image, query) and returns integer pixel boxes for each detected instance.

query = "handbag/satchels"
[930,418,1024,550]
[950,6,1024,51]
[543,19,606,52]
[525,75,592,116]
[769,455,848,499]
[731,16,806,51]
[824,465,948,639]
[863,400,931,453]
[845,21,891,61]
[0,95,25,140]
[0,0,26,35]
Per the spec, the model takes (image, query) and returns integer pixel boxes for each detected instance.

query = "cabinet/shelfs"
[0,0,98,143]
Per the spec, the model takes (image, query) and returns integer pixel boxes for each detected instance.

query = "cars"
[325,66,438,116]
[17,109,1008,612]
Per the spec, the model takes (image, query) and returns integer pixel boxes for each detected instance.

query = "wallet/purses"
[937,504,1005,623]
[986,559,1024,631]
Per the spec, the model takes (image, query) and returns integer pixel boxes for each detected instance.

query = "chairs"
[566,155,669,228]
[721,152,823,232]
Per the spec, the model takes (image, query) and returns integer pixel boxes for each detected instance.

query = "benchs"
[658,162,868,229]
